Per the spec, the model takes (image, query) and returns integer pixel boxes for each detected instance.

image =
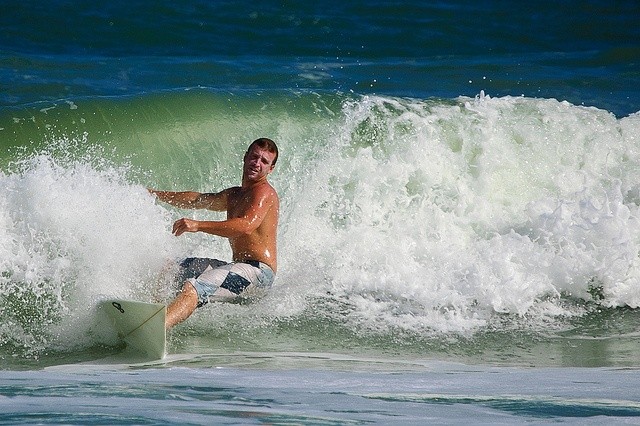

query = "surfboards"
[100,299,166,359]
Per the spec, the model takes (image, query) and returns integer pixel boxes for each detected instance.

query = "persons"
[147,138,279,332]
